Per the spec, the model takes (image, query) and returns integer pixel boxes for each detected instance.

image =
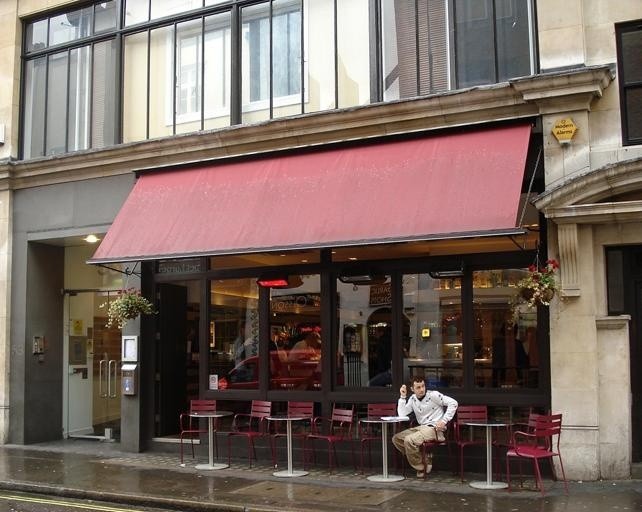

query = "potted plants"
[520,287,555,304]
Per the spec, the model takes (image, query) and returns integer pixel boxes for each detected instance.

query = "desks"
[464,421,514,490]
[408,362,493,388]
[266,412,312,478]
[359,416,410,483]
[187,410,235,471]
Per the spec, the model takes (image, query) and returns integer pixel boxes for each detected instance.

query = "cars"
[218,351,344,389]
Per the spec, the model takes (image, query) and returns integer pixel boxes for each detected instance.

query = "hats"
[311,332,322,344]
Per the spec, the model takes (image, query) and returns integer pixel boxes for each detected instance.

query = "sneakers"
[416,451,433,478]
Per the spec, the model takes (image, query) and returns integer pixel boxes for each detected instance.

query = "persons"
[391,375,459,479]
[490,319,530,389]
[375,325,393,374]
[287,330,322,383]
[520,325,538,389]
[232,320,258,382]
[439,329,463,387]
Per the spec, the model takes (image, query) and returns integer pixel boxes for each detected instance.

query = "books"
[380,415,410,421]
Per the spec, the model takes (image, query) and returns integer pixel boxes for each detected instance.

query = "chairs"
[496,406,567,493]
[272,401,318,468]
[402,417,450,480]
[228,400,273,469]
[304,401,356,471]
[179,400,220,464]
[357,402,399,470]
[454,404,502,483]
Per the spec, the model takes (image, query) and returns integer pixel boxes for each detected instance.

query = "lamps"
[422,327,431,338]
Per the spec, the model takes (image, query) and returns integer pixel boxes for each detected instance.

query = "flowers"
[105,289,161,328]
[509,259,564,330]
